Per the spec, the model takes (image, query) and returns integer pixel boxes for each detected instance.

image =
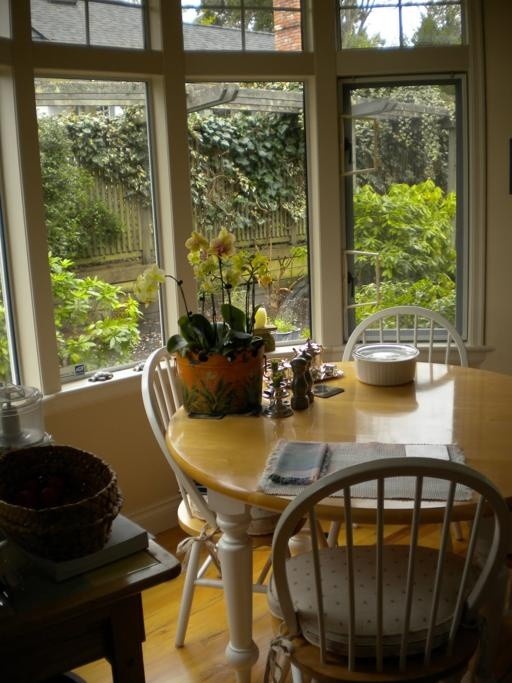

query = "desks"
[1,541,182,682]
[168,361,510,682]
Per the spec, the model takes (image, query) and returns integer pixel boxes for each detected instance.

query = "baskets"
[0,445,124,563]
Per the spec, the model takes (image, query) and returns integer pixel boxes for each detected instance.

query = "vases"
[175,336,265,418]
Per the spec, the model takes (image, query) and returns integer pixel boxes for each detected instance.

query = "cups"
[323,363,338,376]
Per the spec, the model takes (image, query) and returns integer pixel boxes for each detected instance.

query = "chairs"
[263,459,508,682]
[141,346,308,648]
[337,305,470,543]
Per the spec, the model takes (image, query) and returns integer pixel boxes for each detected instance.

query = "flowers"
[132,227,276,361]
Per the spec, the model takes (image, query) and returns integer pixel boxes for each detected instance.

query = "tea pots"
[293,338,325,369]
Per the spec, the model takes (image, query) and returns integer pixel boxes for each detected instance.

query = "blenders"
[1,384,52,459]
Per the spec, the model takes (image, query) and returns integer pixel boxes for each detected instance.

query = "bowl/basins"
[351,342,419,386]
